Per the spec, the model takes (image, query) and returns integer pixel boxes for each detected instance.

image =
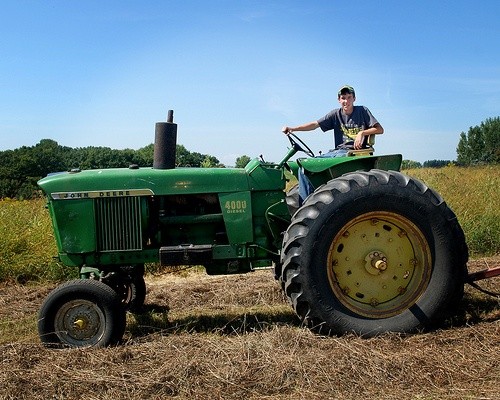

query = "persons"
[280,84,385,208]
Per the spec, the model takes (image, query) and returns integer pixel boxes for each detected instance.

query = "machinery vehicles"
[37,111,469,351]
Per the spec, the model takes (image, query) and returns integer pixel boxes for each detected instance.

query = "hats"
[338,85,354,94]
